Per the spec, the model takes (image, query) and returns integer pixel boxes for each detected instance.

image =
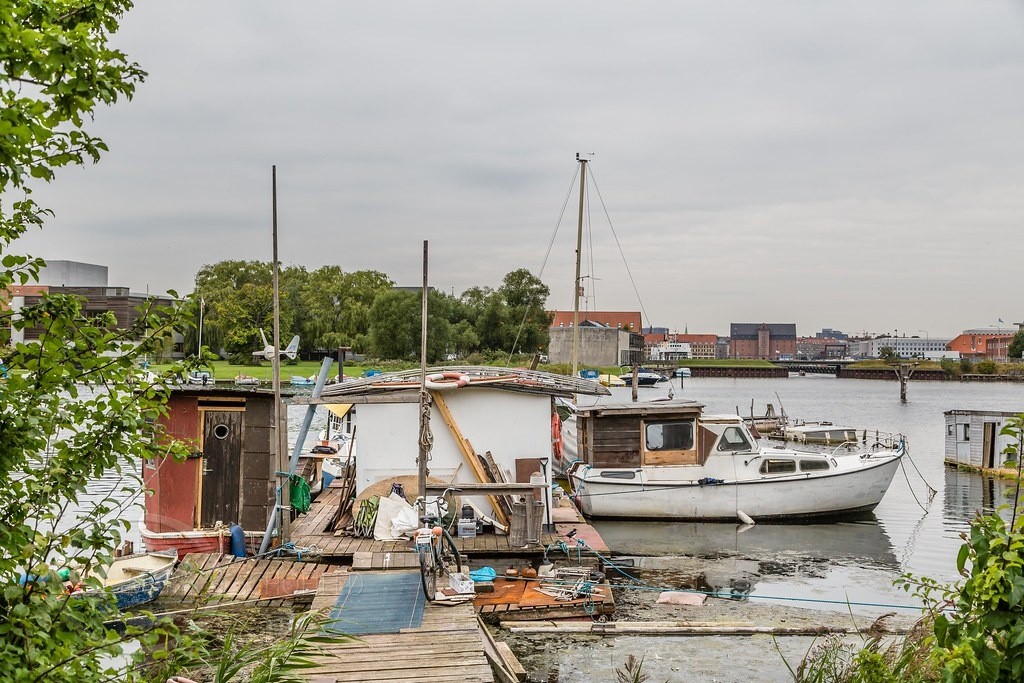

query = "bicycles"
[416,487,462,602]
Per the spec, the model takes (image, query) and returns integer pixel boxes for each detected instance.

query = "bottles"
[522,565,537,578]
[505,564,520,576]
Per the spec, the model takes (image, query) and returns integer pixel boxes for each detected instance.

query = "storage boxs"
[321,458,342,490]
[448,571,475,593]
[458,522,479,538]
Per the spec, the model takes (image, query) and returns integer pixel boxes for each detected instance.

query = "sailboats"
[117,284,215,384]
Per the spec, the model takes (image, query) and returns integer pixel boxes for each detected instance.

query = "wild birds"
[557,528,577,542]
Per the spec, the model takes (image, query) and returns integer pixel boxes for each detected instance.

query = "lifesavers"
[424,371,470,390]
[553,412,562,460]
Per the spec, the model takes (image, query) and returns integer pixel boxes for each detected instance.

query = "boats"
[290,368,383,385]
[676,368,690,378]
[235,375,258,385]
[12,548,178,612]
[512,147,909,521]
[136,384,357,557]
[799,370,805,376]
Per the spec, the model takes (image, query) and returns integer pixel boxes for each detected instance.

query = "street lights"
[990,325,1000,356]
[919,330,928,340]
[895,330,897,354]
